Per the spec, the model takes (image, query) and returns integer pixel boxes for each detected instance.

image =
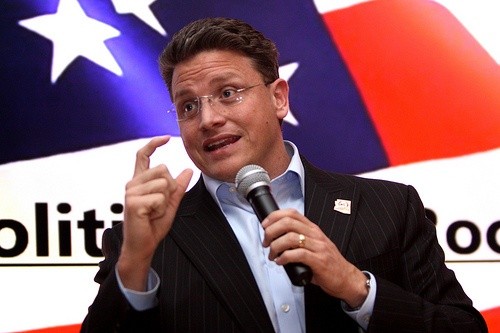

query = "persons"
[76,15,490,332]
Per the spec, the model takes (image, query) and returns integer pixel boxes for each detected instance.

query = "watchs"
[342,271,372,311]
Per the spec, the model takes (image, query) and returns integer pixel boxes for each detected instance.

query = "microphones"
[234,164,312,287]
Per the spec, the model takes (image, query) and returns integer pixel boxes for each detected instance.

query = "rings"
[298,233,308,248]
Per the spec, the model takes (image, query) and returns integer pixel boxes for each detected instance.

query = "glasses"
[167,80,272,122]
